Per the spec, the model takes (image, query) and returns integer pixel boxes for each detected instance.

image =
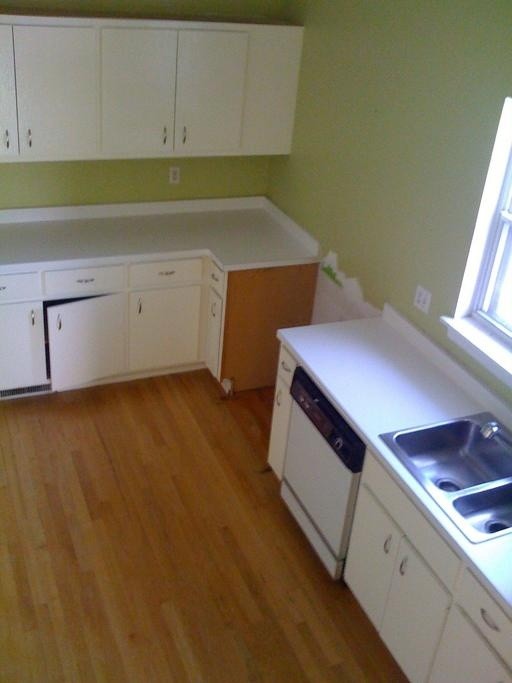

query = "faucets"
[479,418,512,447]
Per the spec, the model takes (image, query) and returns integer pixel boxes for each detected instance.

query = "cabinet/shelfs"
[266,316,363,579]
[0,15,304,164]
[342,448,467,682]
[424,561,511,683]
[0,255,320,401]
[267,342,300,482]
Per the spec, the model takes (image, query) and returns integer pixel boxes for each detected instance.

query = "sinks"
[449,481,512,543]
[378,416,512,495]
